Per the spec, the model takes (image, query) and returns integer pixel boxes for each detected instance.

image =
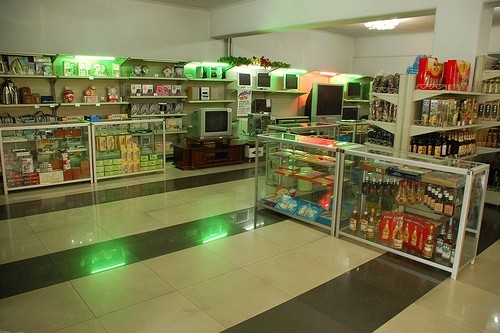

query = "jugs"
[0,78,15,105]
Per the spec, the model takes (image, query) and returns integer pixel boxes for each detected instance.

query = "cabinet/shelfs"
[371,74,407,159]
[0,52,62,124]
[333,144,490,280]
[335,120,370,144]
[400,74,486,176]
[171,137,244,171]
[0,122,93,196]
[121,57,188,158]
[234,61,311,164]
[252,134,365,236]
[470,52,500,204]
[186,59,237,108]
[83,119,166,184]
[52,53,129,122]
[266,121,340,187]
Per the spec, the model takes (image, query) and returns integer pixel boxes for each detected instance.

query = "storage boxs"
[3,56,182,187]
[417,58,471,126]
[261,150,430,228]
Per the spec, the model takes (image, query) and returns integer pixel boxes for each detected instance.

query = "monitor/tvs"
[283,72,300,90]
[257,73,271,90]
[192,108,232,138]
[255,98,272,114]
[305,82,361,124]
[236,72,252,87]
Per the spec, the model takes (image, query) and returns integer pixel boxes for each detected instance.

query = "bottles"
[349,176,456,268]
[410,78,500,159]
